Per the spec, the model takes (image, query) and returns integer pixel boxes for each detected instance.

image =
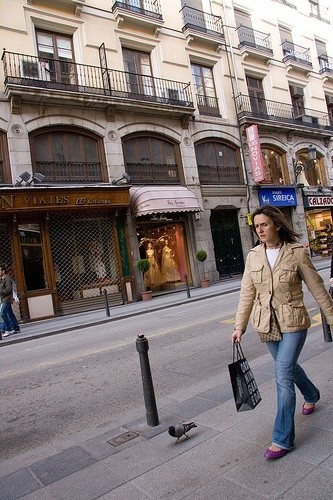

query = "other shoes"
[3,331,12,337]
[264,447,287,459]
[302,402,315,415]
[11,330,20,333]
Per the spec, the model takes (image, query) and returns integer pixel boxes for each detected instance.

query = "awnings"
[130,185,205,218]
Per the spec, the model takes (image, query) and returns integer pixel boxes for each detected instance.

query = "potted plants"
[196,250,210,288]
[138,259,152,300]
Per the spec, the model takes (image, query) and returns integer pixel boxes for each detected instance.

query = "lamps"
[112,173,130,185]
[317,187,324,193]
[251,184,261,190]
[15,172,31,186]
[292,144,317,183]
[26,173,45,187]
[328,186,333,191]
[296,183,304,189]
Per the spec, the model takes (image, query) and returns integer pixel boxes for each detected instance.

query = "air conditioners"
[163,88,187,104]
[19,60,50,81]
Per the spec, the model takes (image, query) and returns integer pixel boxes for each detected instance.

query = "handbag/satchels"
[228,338,262,412]
[258,307,283,343]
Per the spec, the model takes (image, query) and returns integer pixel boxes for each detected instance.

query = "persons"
[0,265,20,338]
[144,240,181,288]
[296,162,311,186]
[231,204,333,460]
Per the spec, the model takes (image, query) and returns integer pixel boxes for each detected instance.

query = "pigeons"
[167,421,197,444]
